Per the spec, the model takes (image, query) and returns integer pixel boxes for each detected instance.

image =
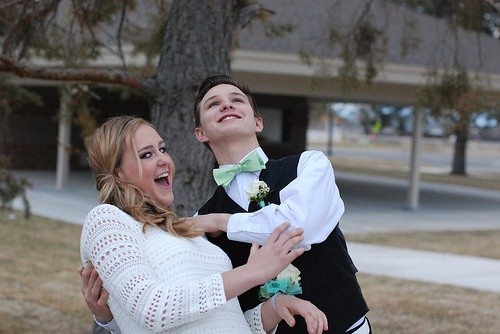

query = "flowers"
[244,180,271,208]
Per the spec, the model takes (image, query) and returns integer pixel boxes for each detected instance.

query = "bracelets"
[258,262,303,310]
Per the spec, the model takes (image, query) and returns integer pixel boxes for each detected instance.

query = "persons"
[76,74,373,333]
[78,115,330,334]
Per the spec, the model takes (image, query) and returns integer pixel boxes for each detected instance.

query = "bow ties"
[213,150,266,187]
